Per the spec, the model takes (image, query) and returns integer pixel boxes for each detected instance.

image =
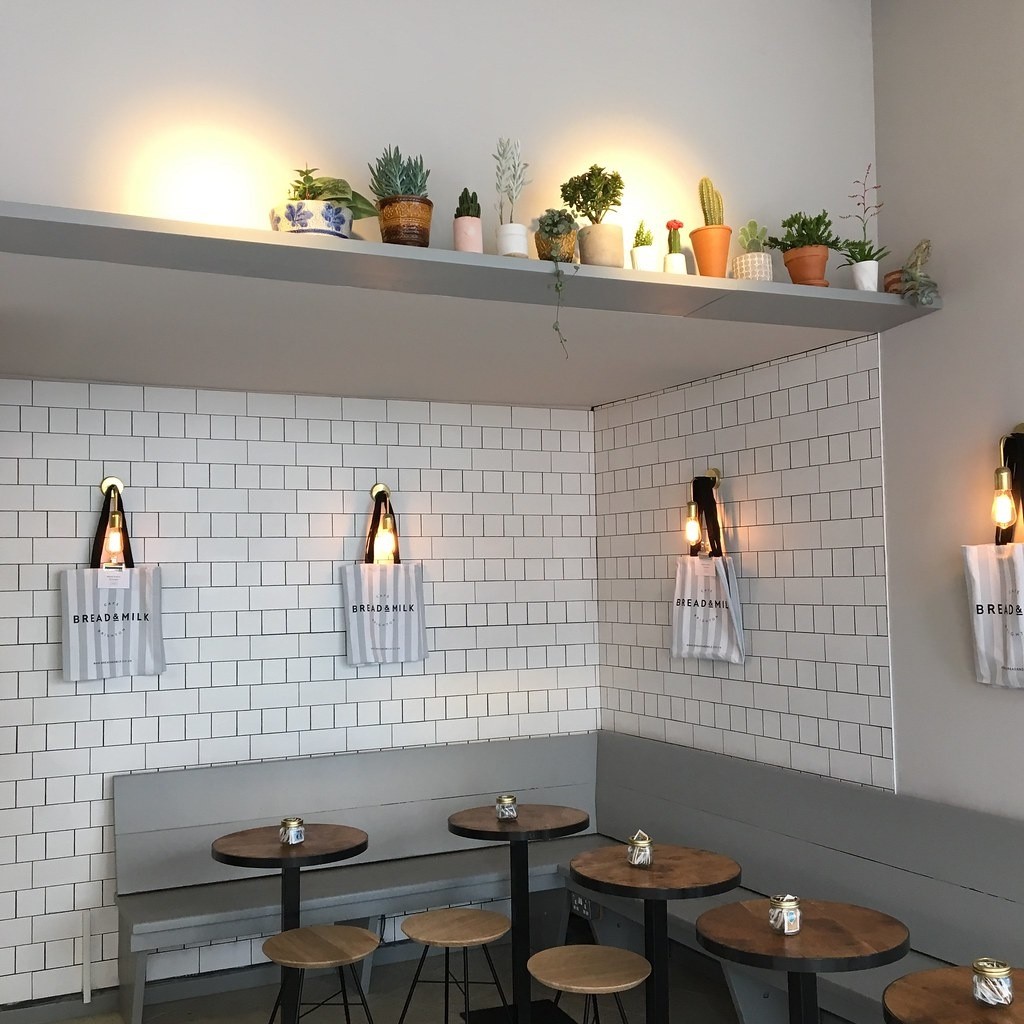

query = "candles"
[689,175,732,278]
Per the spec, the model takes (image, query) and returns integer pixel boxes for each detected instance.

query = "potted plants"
[491,136,533,258]
[269,163,380,239]
[731,218,773,282]
[535,206,579,263]
[368,144,433,247]
[666,219,687,274]
[560,163,625,267]
[836,163,893,292]
[764,209,842,287]
[884,238,940,309]
[630,220,657,272]
[453,188,483,253]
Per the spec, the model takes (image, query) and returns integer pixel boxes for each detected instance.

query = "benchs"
[597,730,1024,1024]
[112,731,598,1024]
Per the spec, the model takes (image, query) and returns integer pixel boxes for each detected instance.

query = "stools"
[402,908,509,1024]
[525,946,652,1024]
[263,927,382,1024]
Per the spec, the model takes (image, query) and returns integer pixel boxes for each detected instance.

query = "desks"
[212,804,1023,1024]
